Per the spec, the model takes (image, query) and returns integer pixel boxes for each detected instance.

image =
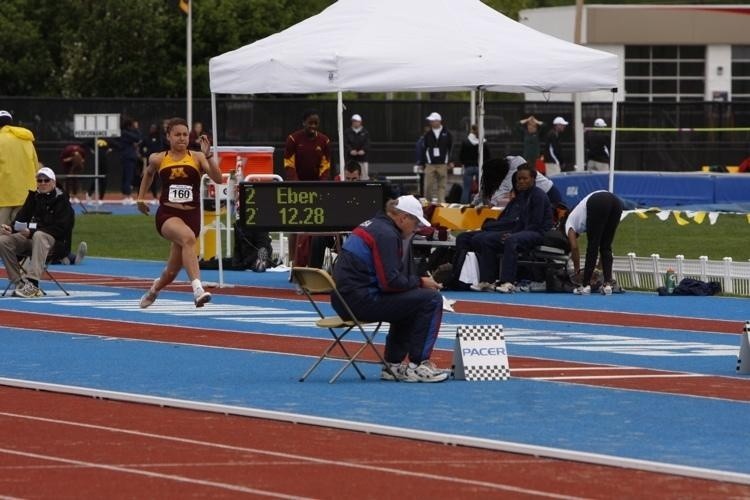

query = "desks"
[283,231,353,282]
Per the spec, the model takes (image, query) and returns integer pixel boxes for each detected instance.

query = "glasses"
[37,178,51,182]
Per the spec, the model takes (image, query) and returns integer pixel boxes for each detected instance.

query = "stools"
[2,250,70,296]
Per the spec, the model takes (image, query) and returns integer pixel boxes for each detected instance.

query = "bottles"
[666,269,677,293]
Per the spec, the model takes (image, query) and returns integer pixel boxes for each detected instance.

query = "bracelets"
[205,152,213,160]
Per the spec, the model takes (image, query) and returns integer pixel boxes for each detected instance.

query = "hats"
[393,194,431,228]
[35,166,56,182]
[553,116,569,125]
[593,118,608,127]
[0,110,13,120]
[426,112,441,121]
[351,114,362,122]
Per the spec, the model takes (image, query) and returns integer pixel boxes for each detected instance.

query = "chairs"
[292,267,400,384]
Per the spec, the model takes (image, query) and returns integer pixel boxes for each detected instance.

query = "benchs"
[412,207,573,287]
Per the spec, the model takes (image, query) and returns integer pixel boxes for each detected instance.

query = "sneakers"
[194,291,212,307]
[139,277,160,308]
[470,281,517,294]
[11,280,45,297]
[380,359,449,383]
[573,285,591,295]
[57,241,87,265]
[599,284,612,295]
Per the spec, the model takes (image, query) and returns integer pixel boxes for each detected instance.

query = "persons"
[0,110,38,258]
[413,113,621,296]
[62,120,208,208]
[0,167,72,298]
[329,194,454,384]
[285,113,368,180]
[135,119,223,307]
